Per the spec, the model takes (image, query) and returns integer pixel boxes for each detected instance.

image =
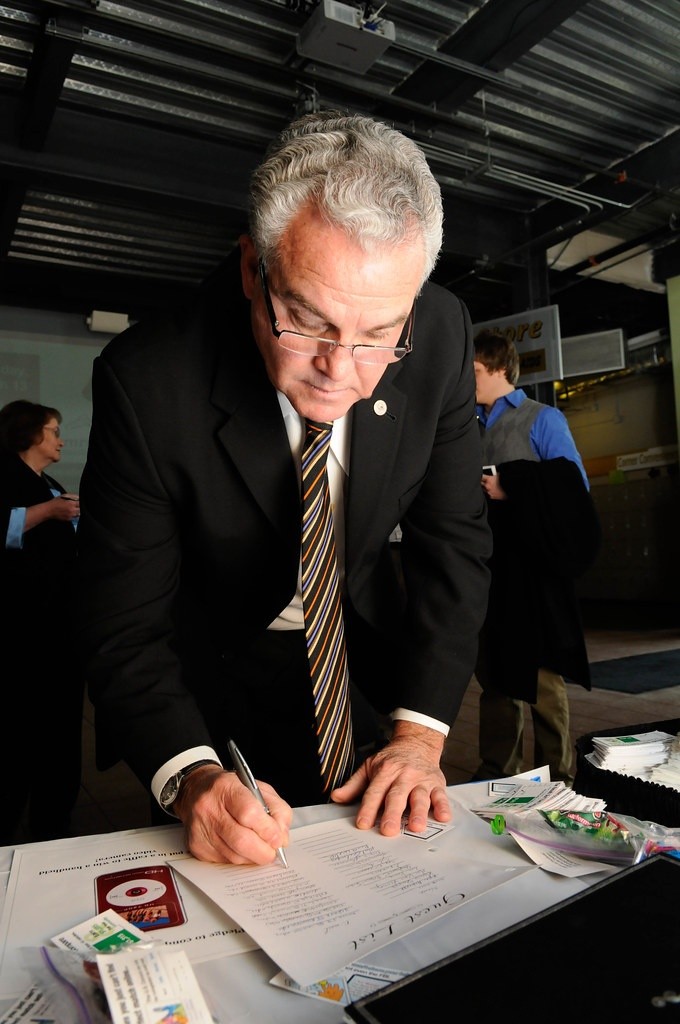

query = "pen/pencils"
[228,739,288,868]
[60,496,77,501]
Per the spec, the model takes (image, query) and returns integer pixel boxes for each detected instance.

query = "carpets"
[560,647,680,695]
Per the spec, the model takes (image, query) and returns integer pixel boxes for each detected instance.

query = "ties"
[302,417,355,799]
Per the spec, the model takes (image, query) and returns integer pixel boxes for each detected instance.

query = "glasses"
[43,426,60,438]
[256,256,417,363]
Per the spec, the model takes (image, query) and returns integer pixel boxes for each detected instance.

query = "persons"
[77,108,493,868]
[0,400,81,845]
[470,331,592,781]
